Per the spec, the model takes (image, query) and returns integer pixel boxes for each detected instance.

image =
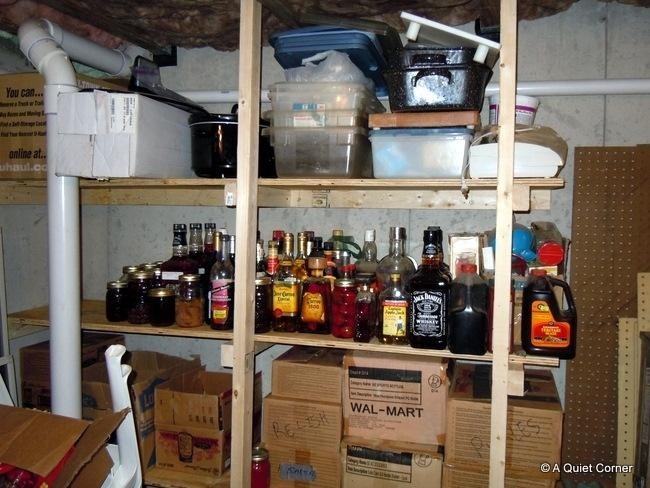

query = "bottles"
[163,222,452,350]
[530,221,564,265]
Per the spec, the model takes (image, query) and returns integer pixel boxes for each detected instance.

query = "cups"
[179,432,192,462]
[488,91,540,126]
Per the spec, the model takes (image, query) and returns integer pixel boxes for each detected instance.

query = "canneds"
[250,445,271,487]
[330,279,355,339]
[255,278,273,334]
[106,262,205,328]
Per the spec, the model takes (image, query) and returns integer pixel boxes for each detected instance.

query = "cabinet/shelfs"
[0,1,567,488]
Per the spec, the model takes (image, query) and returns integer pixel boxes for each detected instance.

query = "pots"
[187,104,274,178]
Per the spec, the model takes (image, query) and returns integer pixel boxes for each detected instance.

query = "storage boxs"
[0,72,129,181]
[260,80,387,179]
[57,93,194,179]
[368,128,473,179]
[385,42,493,112]
